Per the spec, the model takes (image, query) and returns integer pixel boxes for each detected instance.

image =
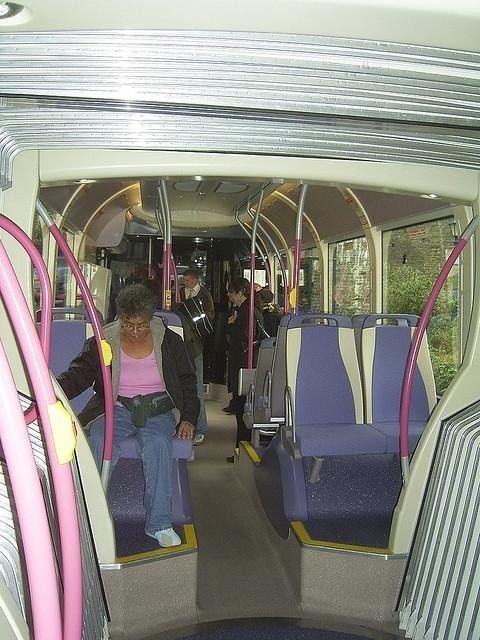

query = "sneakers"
[192,433,206,446]
[139,526,184,549]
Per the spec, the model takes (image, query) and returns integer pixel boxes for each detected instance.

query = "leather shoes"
[222,406,235,414]
[226,455,234,463]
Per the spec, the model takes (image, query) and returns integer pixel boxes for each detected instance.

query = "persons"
[171,296,209,446]
[223,285,239,416]
[55,284,201,548]
[225,277,264,463]
[173,269,216,321]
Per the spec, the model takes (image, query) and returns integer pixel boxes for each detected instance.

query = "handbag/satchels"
[238,307,270,354]
[115,391,175,428]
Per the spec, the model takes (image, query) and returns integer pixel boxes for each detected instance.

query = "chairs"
[284,314,387,457]
[361,314,438,454]
[352,312,386,384]
[112,312,193,460]
[242,336,284,429]
[262,316,359,421]
[35,307,104,418]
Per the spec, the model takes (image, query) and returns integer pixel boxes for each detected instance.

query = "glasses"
[119,323,150,332]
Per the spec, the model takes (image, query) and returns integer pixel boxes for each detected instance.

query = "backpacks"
[175,296,215,338]
